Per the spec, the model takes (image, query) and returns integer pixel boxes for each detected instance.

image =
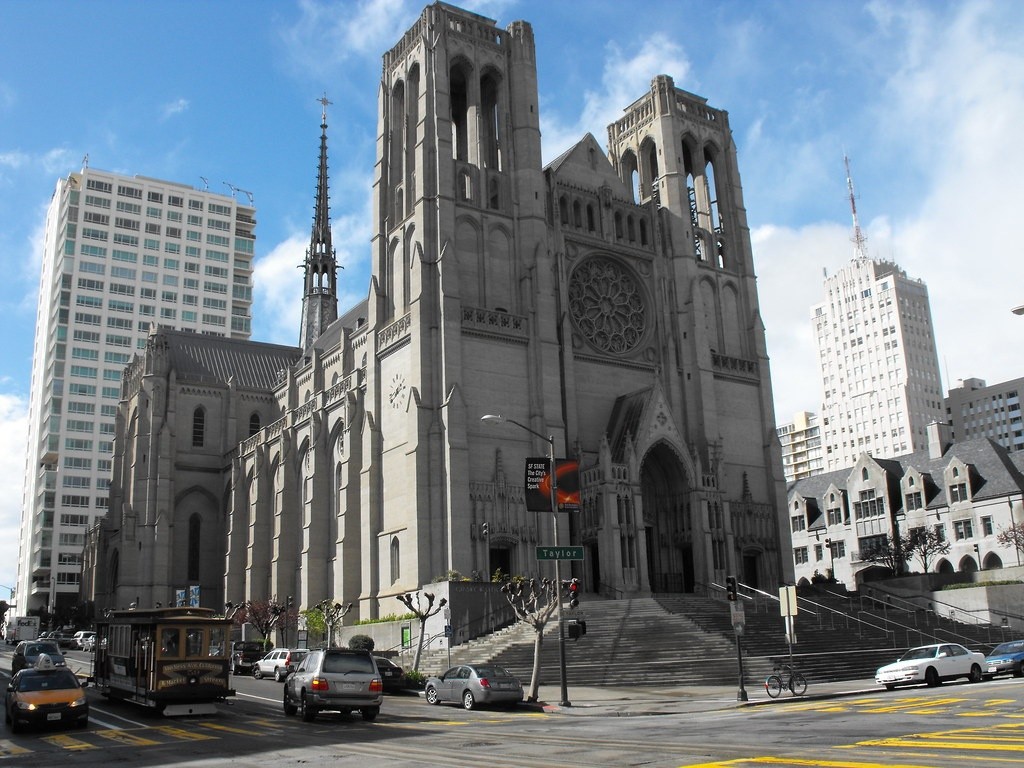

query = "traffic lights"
[574,620,587,635]
[725,576,737,602]
[568,578,581,609]
[569,625,583,639]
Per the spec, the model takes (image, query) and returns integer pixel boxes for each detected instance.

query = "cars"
[424,663,525,711]
[873,642,990,689]
[5,653,91,734]
[373,654,407,694]
[39,629,107,653]
[981,639,1024,681]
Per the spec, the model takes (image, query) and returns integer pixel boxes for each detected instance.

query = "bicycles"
[765,666,808,699]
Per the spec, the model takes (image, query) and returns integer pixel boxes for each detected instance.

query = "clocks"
[389,374,406,409]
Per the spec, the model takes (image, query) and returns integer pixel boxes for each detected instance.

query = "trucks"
[4,616,41,645]
[230,639,268,677]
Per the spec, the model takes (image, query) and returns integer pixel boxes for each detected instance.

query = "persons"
[12,652,28,678]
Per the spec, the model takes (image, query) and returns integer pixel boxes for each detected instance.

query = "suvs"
[252,648,311,682]
[10,638,68,680]
[282,646,385,723]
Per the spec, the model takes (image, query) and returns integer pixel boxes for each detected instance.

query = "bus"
[86,598,238,717]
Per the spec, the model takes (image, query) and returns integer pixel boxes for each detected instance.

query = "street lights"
[50,576,55,631]
[825,538,835,579]
[973,544,982,571]
[284,595,294,648]
[62,554,92,604]
[480,412,572,708]
[482,521,491,583]
